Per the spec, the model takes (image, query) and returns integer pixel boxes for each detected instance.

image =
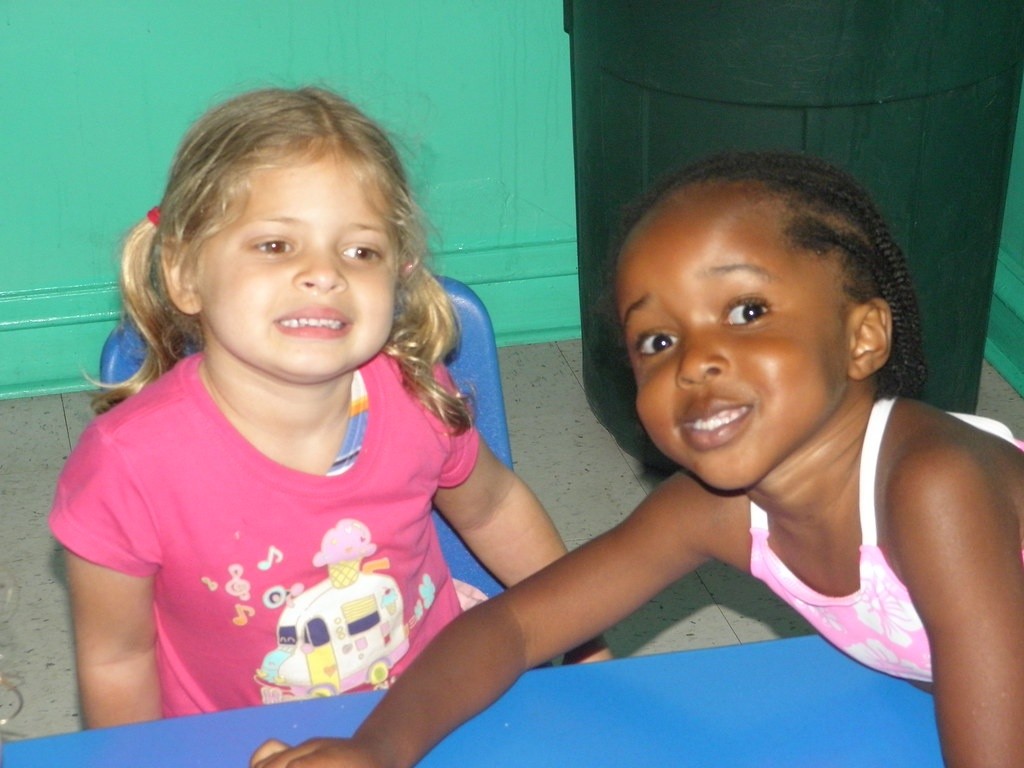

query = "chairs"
[99,273,509,600]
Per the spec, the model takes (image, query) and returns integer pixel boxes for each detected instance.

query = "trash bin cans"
[564,0,1024,473]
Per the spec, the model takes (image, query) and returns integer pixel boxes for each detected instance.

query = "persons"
[251,149,1023,768]
[46,88,612,732]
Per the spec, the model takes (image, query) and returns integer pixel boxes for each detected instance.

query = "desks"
[0,638,944,768]
